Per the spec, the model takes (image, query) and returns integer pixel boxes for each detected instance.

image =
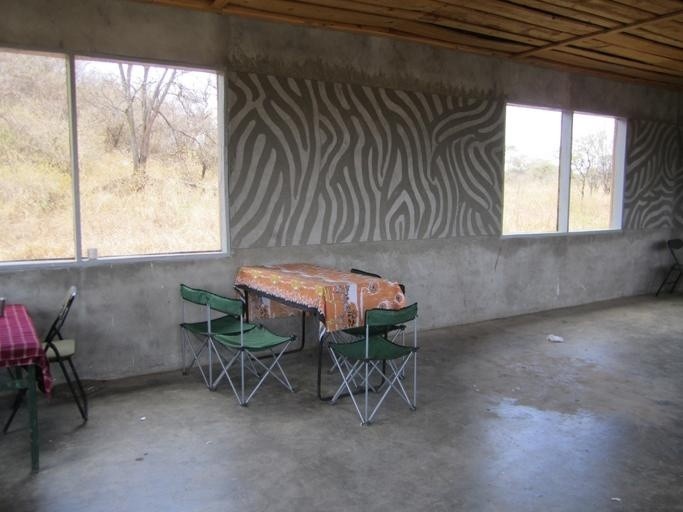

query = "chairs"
[313,269,405,380]
[655,239,683,297]
[3,286,88,433]
[209,292,297,407]
[326,303,420,426]
[179,284,259,378]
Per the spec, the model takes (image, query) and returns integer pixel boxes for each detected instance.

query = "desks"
[0,303,42,472]
[233,263,405,401]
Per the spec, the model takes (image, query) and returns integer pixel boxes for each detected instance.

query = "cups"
[87,247,98,260]
[0,297,6,317]
